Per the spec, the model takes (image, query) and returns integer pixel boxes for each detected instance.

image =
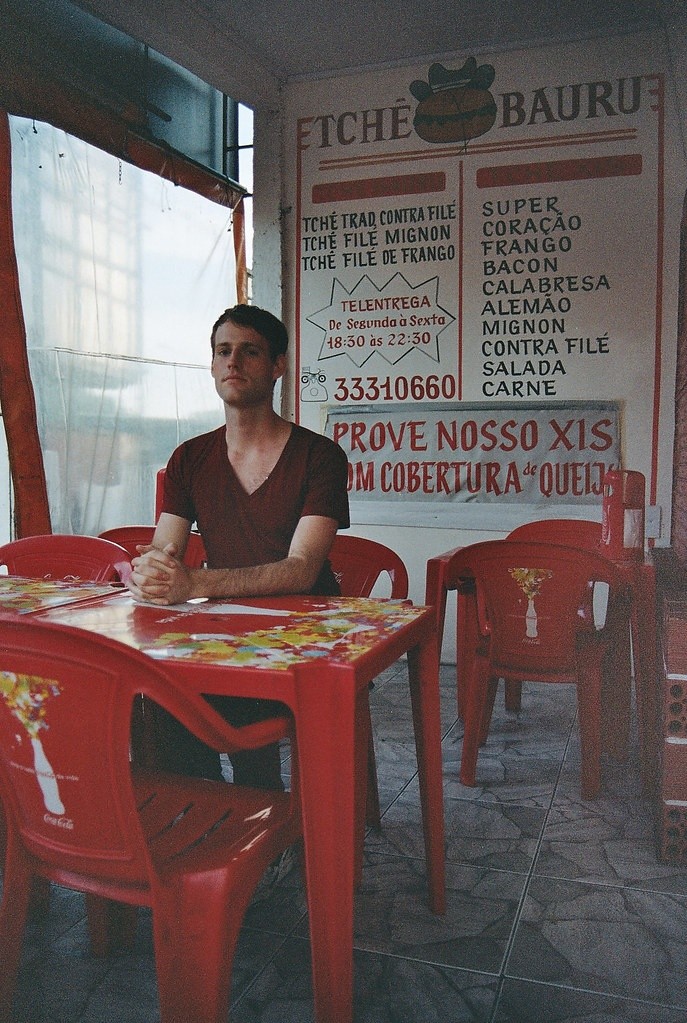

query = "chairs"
[1,514,642,1023]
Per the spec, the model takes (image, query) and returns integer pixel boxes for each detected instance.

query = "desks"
[418,546,655,799]
[0,574,128,622]
[23,587,445,1023]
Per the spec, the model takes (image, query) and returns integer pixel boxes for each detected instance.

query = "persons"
[129,305,352,907]
